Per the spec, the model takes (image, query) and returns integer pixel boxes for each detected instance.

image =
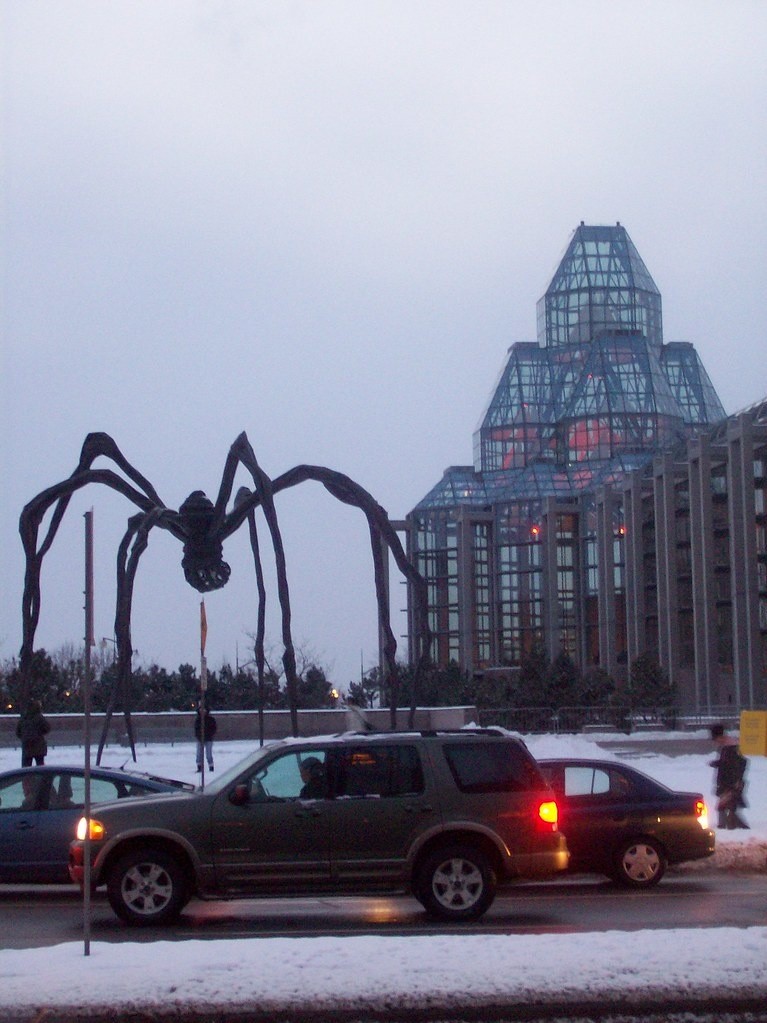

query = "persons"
[22,775,58,807]
[345,697,379,761]
[709,724,750,829]
[299,757,325,800]
[195,705,217,772]
[16,700,50,767]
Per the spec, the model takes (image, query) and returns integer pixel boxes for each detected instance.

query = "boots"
[196,765,202,773]
[209,766,214,772]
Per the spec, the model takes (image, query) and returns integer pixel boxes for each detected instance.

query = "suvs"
[70,728,571,921]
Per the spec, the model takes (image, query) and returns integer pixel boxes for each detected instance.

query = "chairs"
[20,780,73,808]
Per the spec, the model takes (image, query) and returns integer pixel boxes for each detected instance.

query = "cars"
[0,764,197,885]
[535,759,716,887]
